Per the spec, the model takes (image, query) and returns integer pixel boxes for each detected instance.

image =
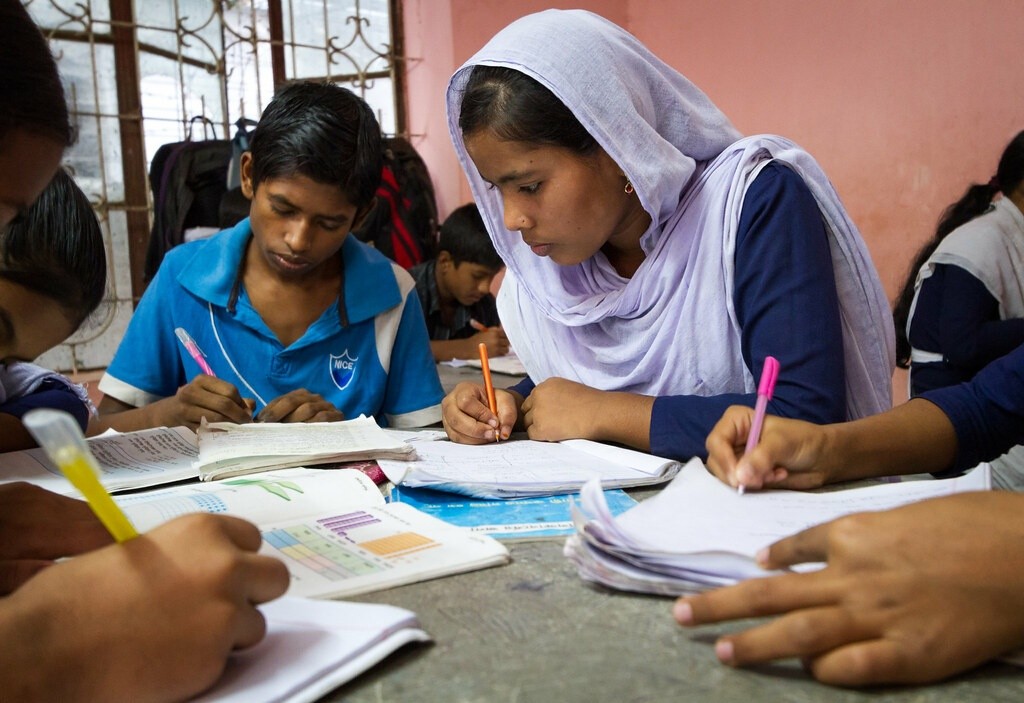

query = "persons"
[0,478,292,703]
[439,9,896,463]
[673,346,1024,681]
[892,129,1024,478]
[1,168,104,375]
[87,79,446,432]
[403,203,509,360]
[0,0,76,230]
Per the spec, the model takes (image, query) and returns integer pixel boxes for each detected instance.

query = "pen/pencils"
[478,342,500,443]
[21,408,139,543]
[174,326,218,379]
[737,355,779,495]
[470,318,488,333]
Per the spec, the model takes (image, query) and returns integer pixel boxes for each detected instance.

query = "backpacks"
[142,115,229,285]
[218,117,258,235]
[358,134,441,278]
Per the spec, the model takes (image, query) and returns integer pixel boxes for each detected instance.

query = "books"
[107,468,509,603]
[387,483,640,541]
[1,425,199,501]
